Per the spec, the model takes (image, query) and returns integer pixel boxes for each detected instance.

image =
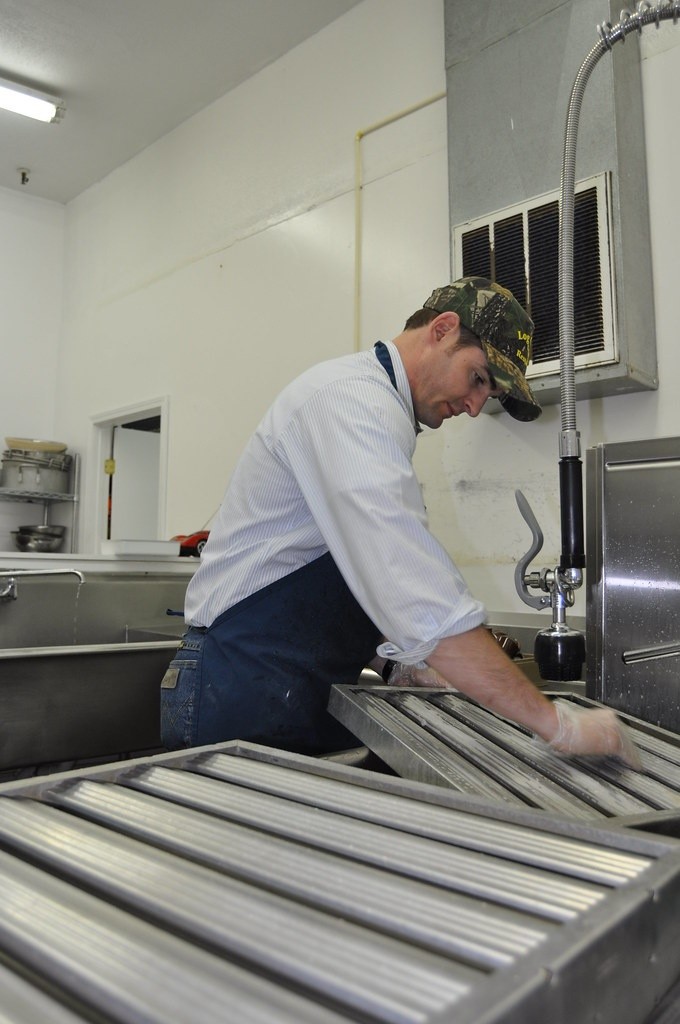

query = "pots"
[0,449,73,493]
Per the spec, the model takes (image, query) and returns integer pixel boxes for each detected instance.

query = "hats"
[424,276,543,422]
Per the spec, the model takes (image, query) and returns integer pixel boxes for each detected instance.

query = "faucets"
[0,566,88,606]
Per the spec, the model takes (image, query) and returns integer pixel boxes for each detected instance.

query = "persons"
[159,277,648,770]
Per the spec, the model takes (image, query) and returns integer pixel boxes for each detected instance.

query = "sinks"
[0,629,186,770]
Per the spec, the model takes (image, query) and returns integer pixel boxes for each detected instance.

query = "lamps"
[0,78,63,126]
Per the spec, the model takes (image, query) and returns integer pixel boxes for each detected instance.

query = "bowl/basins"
[5,438,68,452]
[10,522,67,552]
